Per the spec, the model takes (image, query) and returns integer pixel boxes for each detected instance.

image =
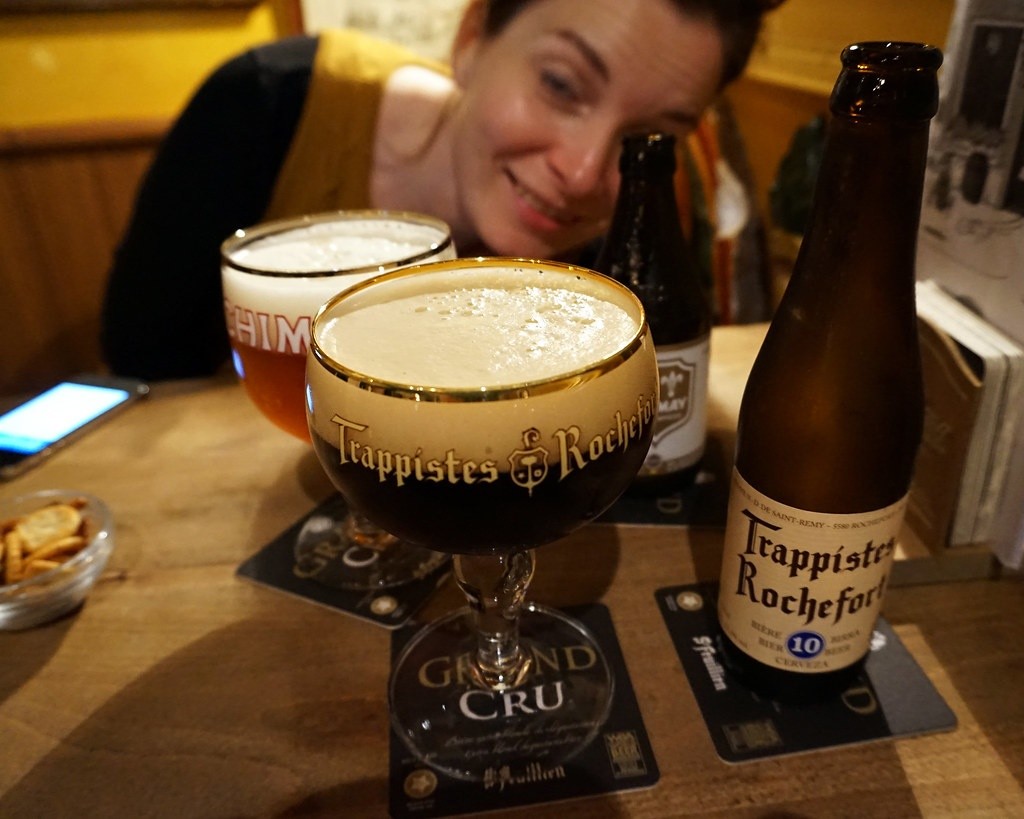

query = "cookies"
[1,497,84,591]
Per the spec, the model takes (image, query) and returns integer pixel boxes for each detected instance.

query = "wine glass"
[310,250,664,784]
[215,210,468,597]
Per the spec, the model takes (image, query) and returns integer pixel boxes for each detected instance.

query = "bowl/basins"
[0,486,122,634]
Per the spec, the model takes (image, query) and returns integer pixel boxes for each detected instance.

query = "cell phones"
[0,372,151,482]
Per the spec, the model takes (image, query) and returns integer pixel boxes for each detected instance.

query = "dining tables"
[0,299,1022,819]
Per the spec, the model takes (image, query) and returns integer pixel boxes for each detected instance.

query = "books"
[914,277,1022,547]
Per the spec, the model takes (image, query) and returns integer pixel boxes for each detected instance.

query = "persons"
[102,0,784,399]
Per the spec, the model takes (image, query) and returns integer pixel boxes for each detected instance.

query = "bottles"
[710,40,941,703]
[591,123,712,499]
